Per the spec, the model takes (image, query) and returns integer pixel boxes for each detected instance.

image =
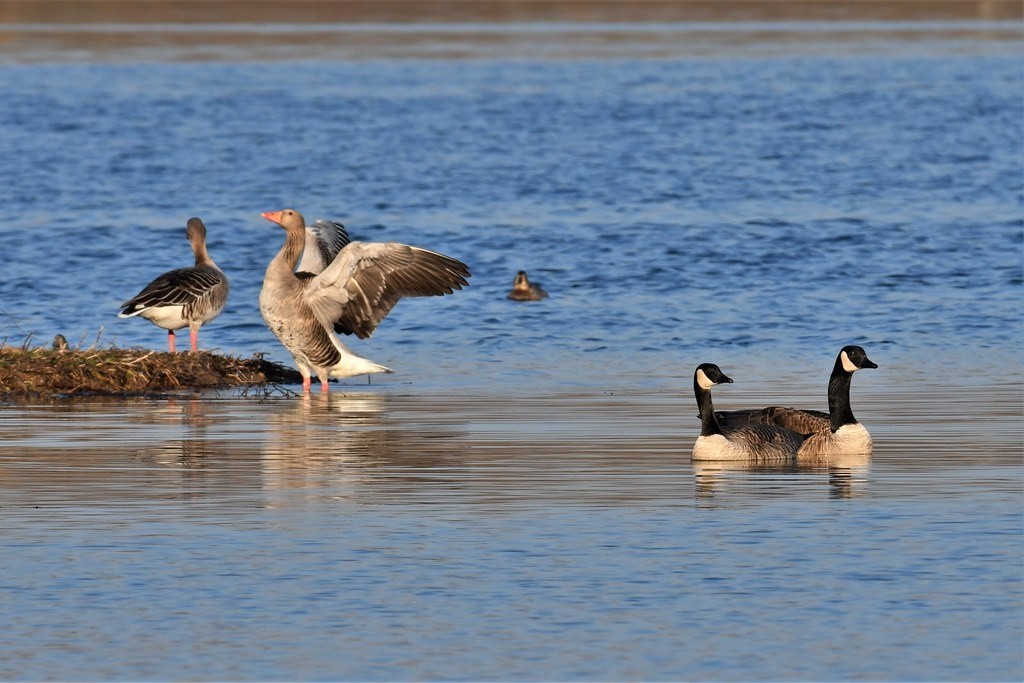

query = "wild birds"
[52,334,69,351]
[691,362,817,459]
[695,344,879,458]
[506,271,550,302]
[119,217,230,353]
[260,209,471,393]
